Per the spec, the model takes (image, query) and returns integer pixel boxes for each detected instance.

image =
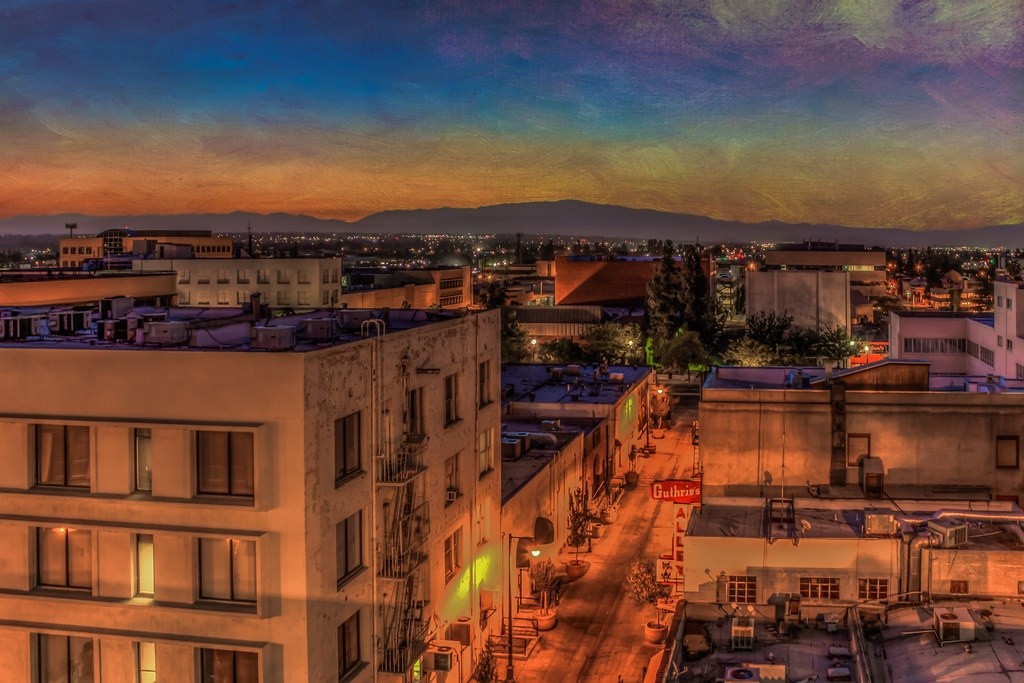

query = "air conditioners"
[453,615,479,646]
[424,640,461,672]
[481,585,502,610]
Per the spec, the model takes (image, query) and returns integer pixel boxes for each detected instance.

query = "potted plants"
[625,445,639,488]
[600,478,618,524]
[622,560,673,644]
[565,487,596,579]
[644,437,656,453]
[652,396,670,439]
[528,558,559,630]
